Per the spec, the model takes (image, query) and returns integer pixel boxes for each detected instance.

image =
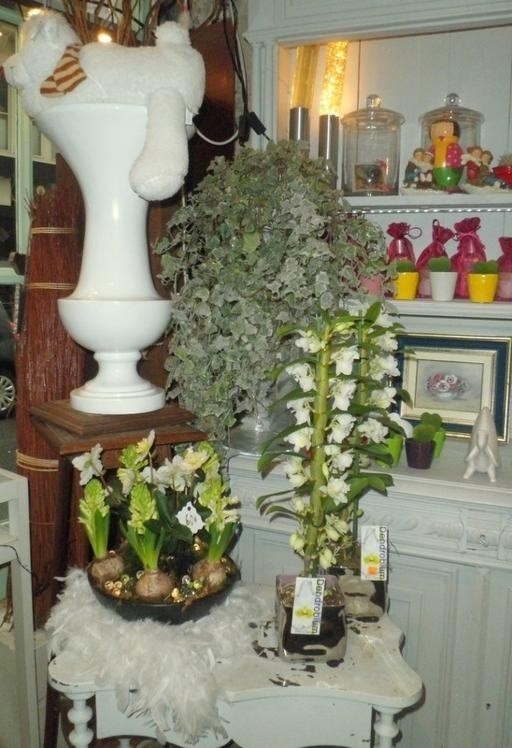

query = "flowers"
[70,428,244,605]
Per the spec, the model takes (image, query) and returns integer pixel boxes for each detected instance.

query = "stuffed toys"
[0,7,208,205]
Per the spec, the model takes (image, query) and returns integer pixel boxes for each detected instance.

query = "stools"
[0,466,43,747]
[25,398,210,568]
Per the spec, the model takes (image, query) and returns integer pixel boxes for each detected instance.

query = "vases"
[87,546,241,626]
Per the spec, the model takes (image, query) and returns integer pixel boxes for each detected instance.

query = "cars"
[0,301,20,419]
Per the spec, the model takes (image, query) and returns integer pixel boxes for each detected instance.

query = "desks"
[44,568,428,748]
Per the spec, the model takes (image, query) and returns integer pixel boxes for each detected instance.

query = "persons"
[400,116,512,196]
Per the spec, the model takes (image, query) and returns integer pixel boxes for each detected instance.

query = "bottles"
[340,92,485,197]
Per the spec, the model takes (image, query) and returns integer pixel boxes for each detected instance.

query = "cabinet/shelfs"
[180,1,511,748]
